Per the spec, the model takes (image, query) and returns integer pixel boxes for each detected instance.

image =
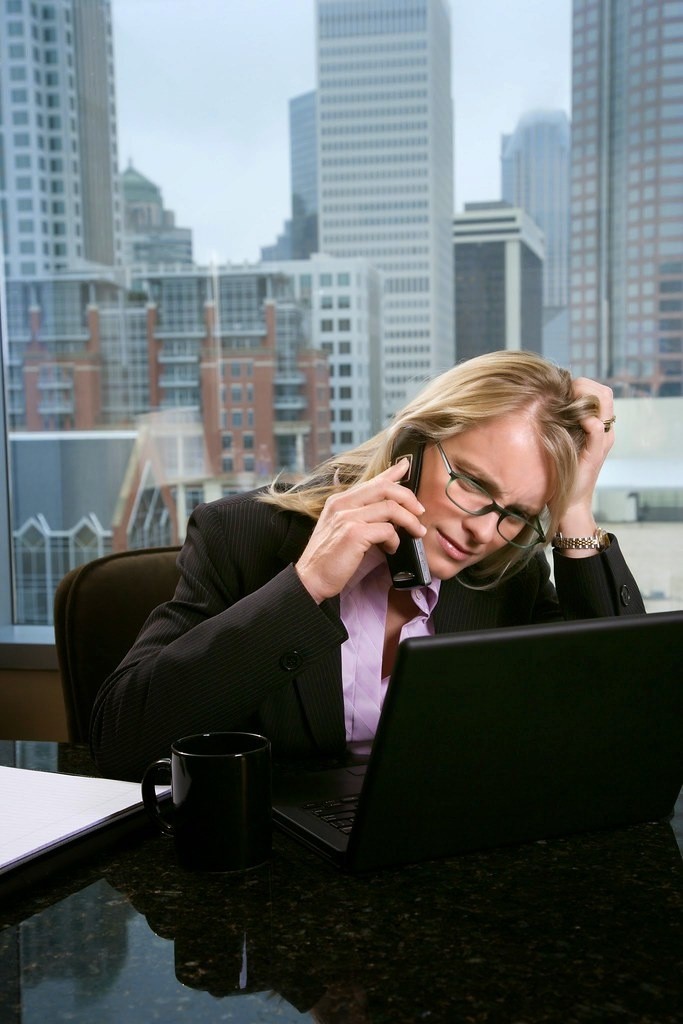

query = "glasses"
[435,441,547,549]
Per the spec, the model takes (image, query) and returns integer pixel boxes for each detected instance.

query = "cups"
[141,732,274,871]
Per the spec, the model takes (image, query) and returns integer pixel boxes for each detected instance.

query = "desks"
[0,739,682,1022]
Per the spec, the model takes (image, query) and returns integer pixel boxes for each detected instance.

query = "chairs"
[54,545,184,744]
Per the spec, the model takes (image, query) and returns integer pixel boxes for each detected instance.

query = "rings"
[604,416,616,431]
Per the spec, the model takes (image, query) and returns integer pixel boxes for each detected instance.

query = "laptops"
[270,611,683,865]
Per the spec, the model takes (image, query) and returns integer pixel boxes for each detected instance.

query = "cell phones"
[385,428,432,591]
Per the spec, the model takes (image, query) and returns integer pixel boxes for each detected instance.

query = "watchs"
[552,529,610,551]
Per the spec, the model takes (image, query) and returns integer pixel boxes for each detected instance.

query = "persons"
[91,351,647,782]
